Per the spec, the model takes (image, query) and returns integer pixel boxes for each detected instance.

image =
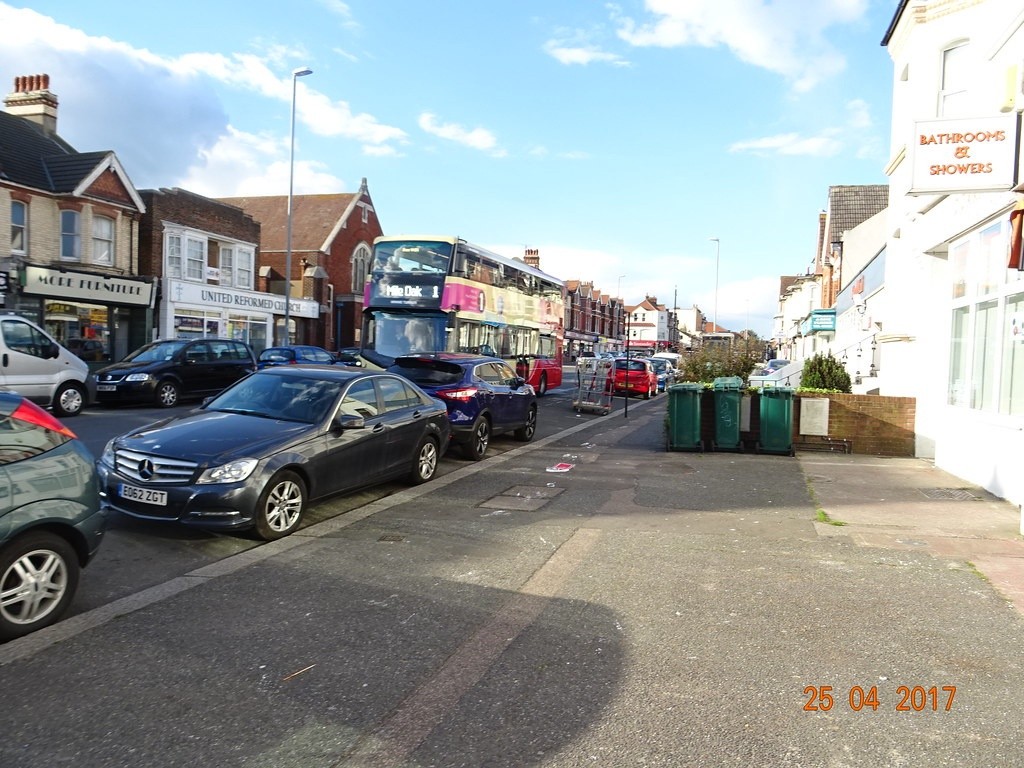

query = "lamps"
[853,294,865,308]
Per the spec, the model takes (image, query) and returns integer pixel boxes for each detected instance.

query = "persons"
[390,323,410,355]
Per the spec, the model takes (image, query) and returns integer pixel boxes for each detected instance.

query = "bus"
[354,235,569,398]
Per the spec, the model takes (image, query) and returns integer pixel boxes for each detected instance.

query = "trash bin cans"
[710,377,744,453]
[666,384,704,453]
[755,386,795,457]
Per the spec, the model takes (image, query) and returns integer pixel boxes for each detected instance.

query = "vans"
[0,311,97,418]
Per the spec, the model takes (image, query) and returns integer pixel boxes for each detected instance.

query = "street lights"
[282,65,314,346]
[710,238,720,334]
[612,274,627,348]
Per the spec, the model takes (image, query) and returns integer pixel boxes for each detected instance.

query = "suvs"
[383,350,539,462]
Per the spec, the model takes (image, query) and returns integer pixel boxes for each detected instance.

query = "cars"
[762,358,790,377]
[576,349,699,393]
[94,363,453,543]
[90,334,259,410]
[607,356,660,399]
[0,387,110,643]
[258,345,340,374]
